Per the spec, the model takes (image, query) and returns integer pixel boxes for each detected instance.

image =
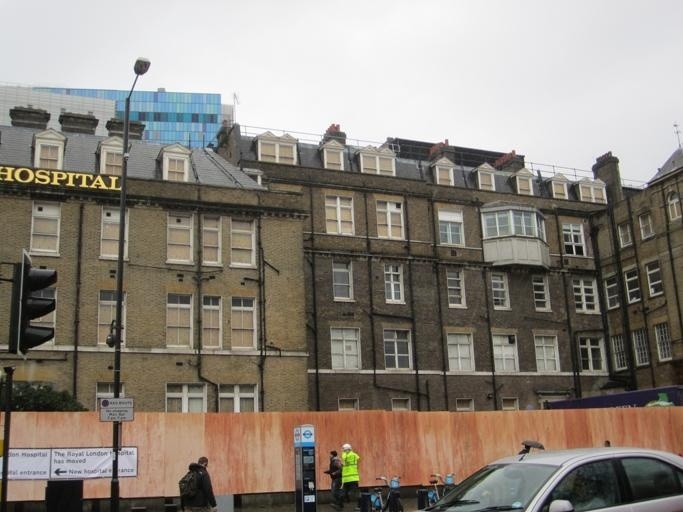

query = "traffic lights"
[7,243,59,359]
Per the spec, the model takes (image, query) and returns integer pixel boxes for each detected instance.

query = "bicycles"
[423,471,455,508]
[356,475,402,512]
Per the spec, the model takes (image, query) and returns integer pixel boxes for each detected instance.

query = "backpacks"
[180,471,197,500]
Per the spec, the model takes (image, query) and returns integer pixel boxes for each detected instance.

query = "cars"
[416,447,682,512]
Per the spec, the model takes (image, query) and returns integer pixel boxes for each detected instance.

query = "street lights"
[102,54,152,512]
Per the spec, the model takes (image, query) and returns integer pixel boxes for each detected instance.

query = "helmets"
[342,444,351,450]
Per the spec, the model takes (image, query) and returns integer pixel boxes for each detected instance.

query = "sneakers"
[330,504,341,511]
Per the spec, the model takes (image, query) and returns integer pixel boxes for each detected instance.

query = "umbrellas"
[520,439,545,452]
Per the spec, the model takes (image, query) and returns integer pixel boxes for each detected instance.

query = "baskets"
[445,475,454,484]
[390,479,400,489]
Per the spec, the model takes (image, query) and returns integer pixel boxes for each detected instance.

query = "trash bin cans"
[44,479,84,512]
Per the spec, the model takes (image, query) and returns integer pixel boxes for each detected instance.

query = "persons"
[177,456,218,511]
[518,444,531,453]
[321,450,350,508]
[328,443,360,511]
[565,466,607,511]
[604,440,610,447]
